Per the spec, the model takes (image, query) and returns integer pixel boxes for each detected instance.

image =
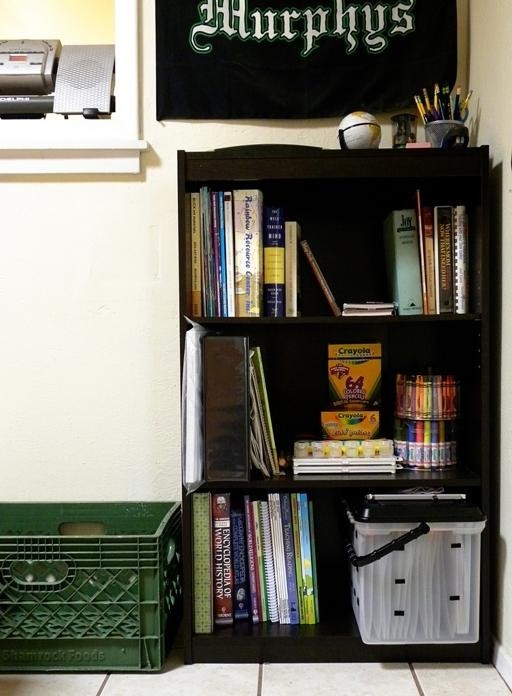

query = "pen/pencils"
[413,83,473,147]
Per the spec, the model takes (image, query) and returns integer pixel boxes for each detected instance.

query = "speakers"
[53,45,114,115]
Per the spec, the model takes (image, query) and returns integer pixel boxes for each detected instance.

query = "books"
[285,220,299,319]
[246,344,281,477]
[263,205,284,317]
[300,239,342,320]
[233,189,262,317]
[243,491,320,626]
[341,300,396,316]
[413,187,470,316]
[189,186,234,317]
[230,507,250,620]
[210,493,234,626]
[390,209,423,314]
[190,492,214,635]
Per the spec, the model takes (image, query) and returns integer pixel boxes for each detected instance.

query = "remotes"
[82,108,101,120]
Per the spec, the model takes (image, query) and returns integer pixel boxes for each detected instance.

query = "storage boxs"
[347,488,488,644]
[0,502,181,674]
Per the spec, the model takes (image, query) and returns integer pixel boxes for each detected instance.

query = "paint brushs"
[396,421,401,466]
[407,421,415,467]
[445,420,452,468]
[396,372,456,420]
[423,420,431,469]
[431,422,440,467]
[415,421,424,469]
[400,419,407,468]
[451,422,456,464]
[437,420,447,469]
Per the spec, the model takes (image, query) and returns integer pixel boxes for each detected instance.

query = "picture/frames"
[0,1,149,174]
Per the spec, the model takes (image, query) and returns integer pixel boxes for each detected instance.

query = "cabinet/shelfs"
[177,144,494,666]
